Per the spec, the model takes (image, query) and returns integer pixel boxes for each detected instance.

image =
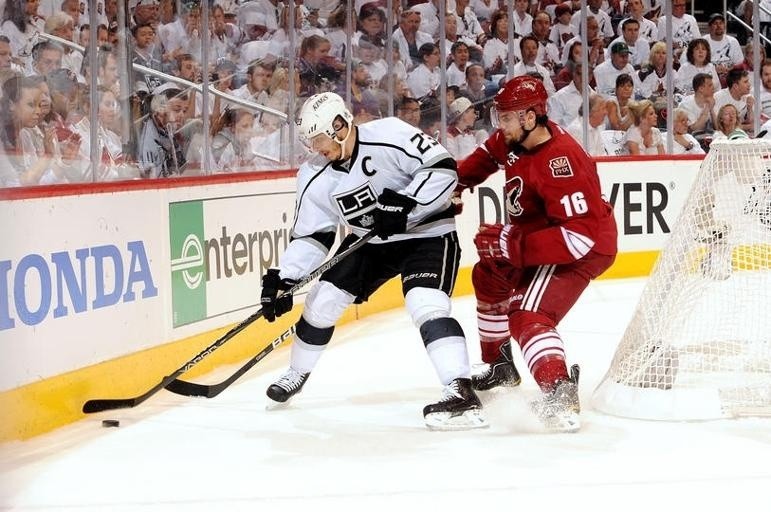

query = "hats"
[48,68,86,90]
[611,42,633,55]
[449,97,472,125]
[708,13,723,24]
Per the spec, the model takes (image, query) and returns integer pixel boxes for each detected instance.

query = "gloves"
[474,224,523,269]
[261,268,296,322]
[373,188,417,240]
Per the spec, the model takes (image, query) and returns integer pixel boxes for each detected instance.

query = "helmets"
[299,92,353,139]
[495,76,548,116]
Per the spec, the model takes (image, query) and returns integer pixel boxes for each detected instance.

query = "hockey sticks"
[162,321,299,399]
[83,230,377,413]
[82,230,376,414]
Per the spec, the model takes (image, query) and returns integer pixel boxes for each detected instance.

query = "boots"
[267,365,311,402]
[424,377,482,418]
[529,364,579,419]
[472,340,520,391]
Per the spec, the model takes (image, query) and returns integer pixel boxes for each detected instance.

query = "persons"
[694,153,771,281]
[0,2,771,191]
[453,74,618,418]
[259,90,483,422]
